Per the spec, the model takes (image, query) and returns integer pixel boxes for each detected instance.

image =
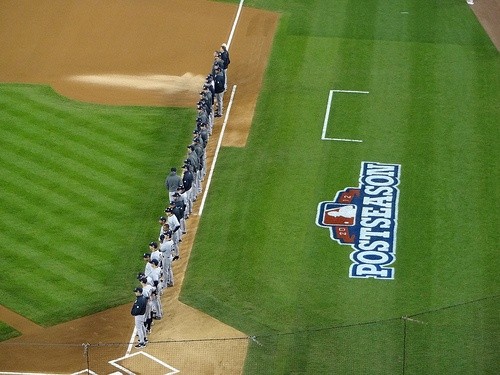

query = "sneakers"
[167,283,174,287]
[135,343,146,349]
[138,337,149,342]
[137,311,164,336]
[172,256,180,261]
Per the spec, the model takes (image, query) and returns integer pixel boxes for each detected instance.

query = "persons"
[132,40,232,350]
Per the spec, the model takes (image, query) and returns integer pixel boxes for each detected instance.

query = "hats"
[149,242,157,248]
[177,185,185,190]
[160,235,165,240]
[143,253,151,258]
[136,272,145,279]
[164,231,171,237]
[192,61,221,142]
[139,277,147,283]
[182,165,188,169]
[170,201,176,205]
[184,159,191,165]
[160,217,166,222]
[150,259,158,264]
[165,207,173,212]
[173,193,181,197]
[188,145,195,151]
[133,287,143,292]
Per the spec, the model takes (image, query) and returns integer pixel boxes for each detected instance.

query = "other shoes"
[184,196,196,219]
[179,240,182,243]
[182,231,186,234]
[214,98,224,117]
